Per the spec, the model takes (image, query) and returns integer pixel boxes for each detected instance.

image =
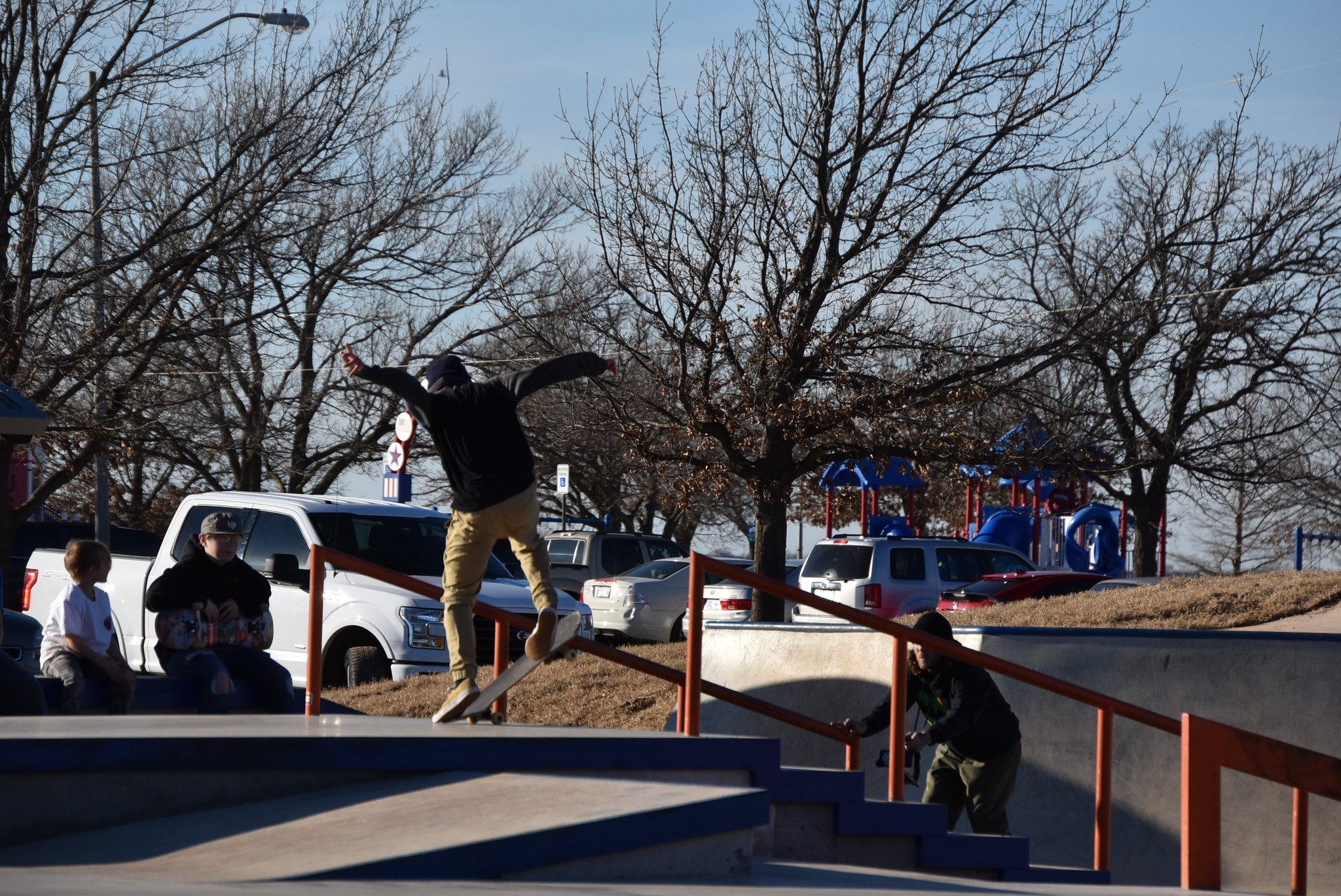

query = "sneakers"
[524,604,558,662]
[431,675,481,725]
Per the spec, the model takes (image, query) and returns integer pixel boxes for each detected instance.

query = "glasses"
[211,534,243,543]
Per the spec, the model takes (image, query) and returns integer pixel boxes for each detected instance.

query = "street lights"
[90,12,311,550]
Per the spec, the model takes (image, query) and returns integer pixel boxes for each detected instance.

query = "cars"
[0,606,44,673]
[1089,577,1173,593]
[577,556,806,643]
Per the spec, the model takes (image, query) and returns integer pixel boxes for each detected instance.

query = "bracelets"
[920,731,934,747]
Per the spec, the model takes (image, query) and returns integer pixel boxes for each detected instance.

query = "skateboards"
[153,604,278,652]
[441,609,582,725]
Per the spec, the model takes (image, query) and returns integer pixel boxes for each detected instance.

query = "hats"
[911,611,954,642]
[200,510,245,536]
[425,355,473,385]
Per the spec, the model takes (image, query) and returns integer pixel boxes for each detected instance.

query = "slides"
[869,498,1141,574]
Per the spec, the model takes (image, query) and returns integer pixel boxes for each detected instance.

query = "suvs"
[935,569,1116,613]
[792,534,1043,624]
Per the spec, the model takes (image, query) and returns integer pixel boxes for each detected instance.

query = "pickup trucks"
[21,491,596,690]
[543,530,690,602]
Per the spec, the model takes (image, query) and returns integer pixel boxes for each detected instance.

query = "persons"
[147,512,295,714]
[830,611,1022,837]
[40,539,137,714]
[340,341,618,722]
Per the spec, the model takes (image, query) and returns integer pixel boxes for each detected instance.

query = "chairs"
[362,525,415,570]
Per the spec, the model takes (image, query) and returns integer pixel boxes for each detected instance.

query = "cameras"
[874,739,920,787]
